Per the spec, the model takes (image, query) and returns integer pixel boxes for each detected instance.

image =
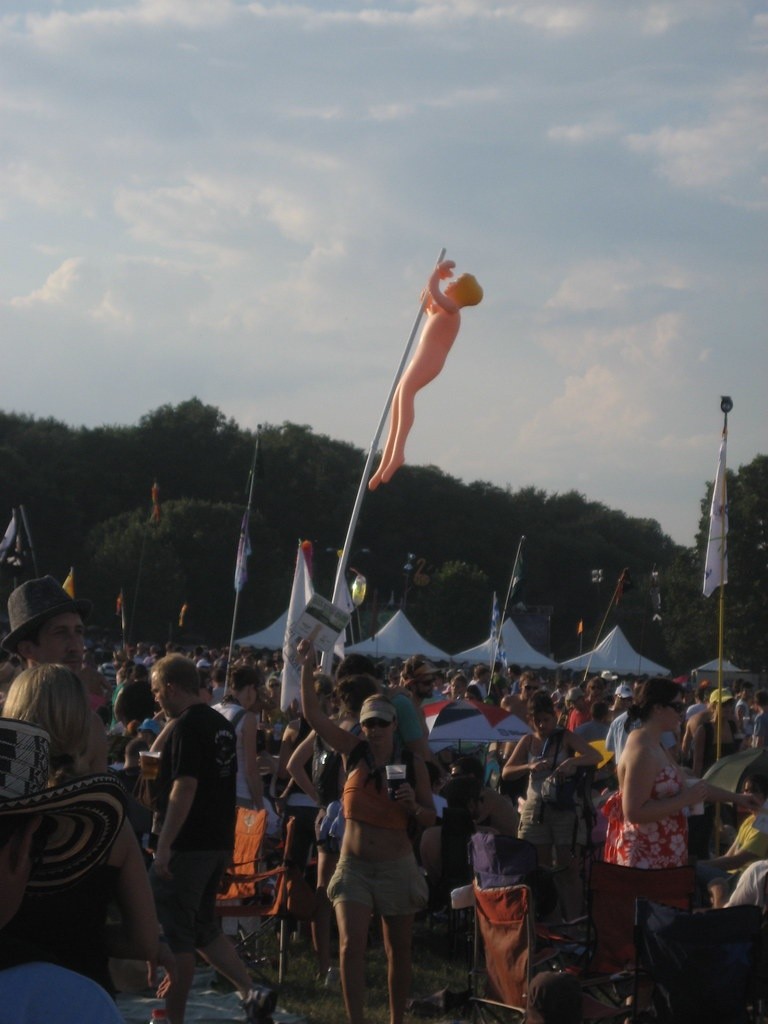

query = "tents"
[562,624,670,677]
[236,609,290,651]
[452,618,563,671]
[343,609,450,662]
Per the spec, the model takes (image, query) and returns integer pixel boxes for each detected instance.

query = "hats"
[137,718,159,737]
[1,717,127,893]
[615,685,633,698]
[359,702,396,722]
[1,575,93,651]
[709,688,735,710]
[602,671,618,680]
[196,660,212,668]
[401,660,442,679]
[566,688,584,701]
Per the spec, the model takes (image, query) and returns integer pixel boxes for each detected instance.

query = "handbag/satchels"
[541,729,591,811]
[683,779,704,816]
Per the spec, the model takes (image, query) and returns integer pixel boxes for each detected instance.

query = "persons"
[2,575,108,777]
[0,717,131,1024]
[368,259,484,490]
[0,641,768,1023]
[1,665,161,1001]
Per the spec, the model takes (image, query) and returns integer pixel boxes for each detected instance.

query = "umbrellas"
[704,748,768,807]
[419,695,532,755]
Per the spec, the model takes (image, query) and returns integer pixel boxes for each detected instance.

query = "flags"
[234,511,252,589]
[510,546,526,597]
[334,567,354,661]
[280,542,318,712]
[704,431,729,597]
[0,510,30,573]
[491,600,507,670]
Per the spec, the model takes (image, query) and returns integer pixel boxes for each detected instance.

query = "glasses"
[668,701,684,713]
[268,683,280,687]
[417,679,435,686]
[363,719,391,729]
[522,683,537,690]
[618,695,632,700]
[588,684,601,690]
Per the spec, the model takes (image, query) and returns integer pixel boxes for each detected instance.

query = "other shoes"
[240,988,280,1024]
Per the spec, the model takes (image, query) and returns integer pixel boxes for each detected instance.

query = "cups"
[686,779,704,814]
[139,752,161,780]
[386,765,406,800]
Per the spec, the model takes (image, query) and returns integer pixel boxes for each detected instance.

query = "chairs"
[423,805,621,1024]
[627,895,768,1024]
[588,856,700,1009]
[208,811,314,990]
[214,804,268,989]
